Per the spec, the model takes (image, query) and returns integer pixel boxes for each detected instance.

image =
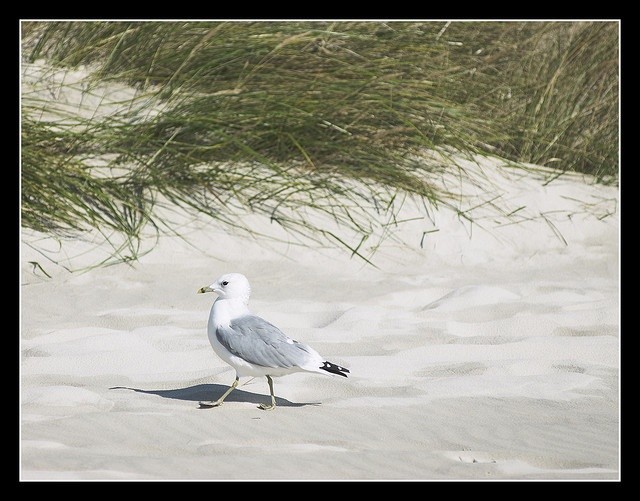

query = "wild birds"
[193,272,349,412]
